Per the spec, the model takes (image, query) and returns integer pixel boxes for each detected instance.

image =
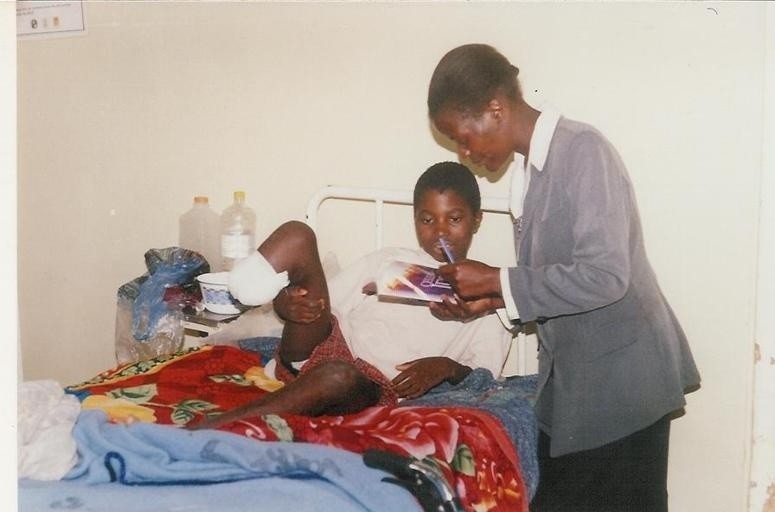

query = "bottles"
[222,190,257,271]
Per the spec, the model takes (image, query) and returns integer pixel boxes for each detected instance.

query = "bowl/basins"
[196,271,253,314]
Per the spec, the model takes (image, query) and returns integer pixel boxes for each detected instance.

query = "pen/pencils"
[439,238,455,264]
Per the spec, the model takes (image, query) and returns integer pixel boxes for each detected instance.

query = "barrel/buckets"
[219,189,256,273]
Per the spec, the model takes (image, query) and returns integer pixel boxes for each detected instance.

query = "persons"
[184,162,514,429]
[428,43,702,511]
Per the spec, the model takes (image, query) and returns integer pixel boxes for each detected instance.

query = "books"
[376,261,458,305]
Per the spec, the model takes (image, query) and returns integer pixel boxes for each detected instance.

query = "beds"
[16,188,537,511]
